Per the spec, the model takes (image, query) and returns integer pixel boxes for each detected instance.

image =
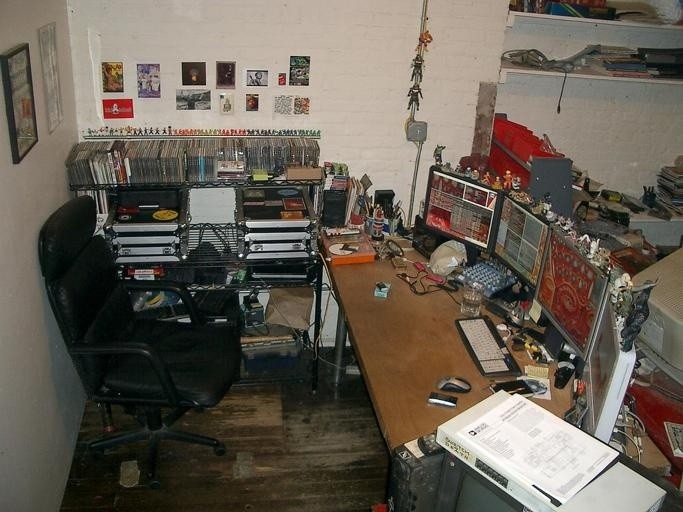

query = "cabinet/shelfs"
[69,130,323,396]
[499,10,683,85]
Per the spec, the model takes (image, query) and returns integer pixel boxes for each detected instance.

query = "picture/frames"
[0,42,38,164]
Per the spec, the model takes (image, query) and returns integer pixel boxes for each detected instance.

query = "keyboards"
[455,315,523,377]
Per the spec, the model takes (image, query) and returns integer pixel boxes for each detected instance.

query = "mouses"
[437,375,472,393]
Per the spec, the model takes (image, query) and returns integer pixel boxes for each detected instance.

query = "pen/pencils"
[366,196,373,217]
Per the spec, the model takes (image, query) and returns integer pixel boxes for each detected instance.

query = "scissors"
[357,195,367,212]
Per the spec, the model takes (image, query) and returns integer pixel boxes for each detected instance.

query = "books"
[656,166,683,215]
[66,140,320,189]
[436,390,623,510]
[510,0,606,13]
[585,45,652,78]
[77,189,109,213]
[310,175,362,224]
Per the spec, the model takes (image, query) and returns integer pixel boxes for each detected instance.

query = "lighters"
[376,282,387,290]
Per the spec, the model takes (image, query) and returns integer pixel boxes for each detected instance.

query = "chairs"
[38,194,240,456]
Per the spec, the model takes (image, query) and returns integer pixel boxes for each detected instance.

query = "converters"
[417,430,445,457]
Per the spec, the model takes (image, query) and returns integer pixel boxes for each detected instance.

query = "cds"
[329,243,353,256]
[524,379,547,395]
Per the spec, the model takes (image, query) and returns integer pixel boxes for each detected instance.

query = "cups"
[459,286,484,319]
[347,221,365,231]
[553,360,576,390]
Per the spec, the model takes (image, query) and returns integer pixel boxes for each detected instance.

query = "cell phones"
[428,392,458,407]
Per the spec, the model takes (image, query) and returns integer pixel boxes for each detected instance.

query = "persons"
[247,97,257,110]
[102,65,122,91]
[249,71,262,85]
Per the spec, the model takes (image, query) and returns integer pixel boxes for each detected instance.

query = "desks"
[321,227,671,479]
[630,201,682,246]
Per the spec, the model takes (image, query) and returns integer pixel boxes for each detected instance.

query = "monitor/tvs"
[582,282,637,446]
[435,450,533,512]
[485,191,550,303]
[422,165,502,268]
[523,222,610,389]
